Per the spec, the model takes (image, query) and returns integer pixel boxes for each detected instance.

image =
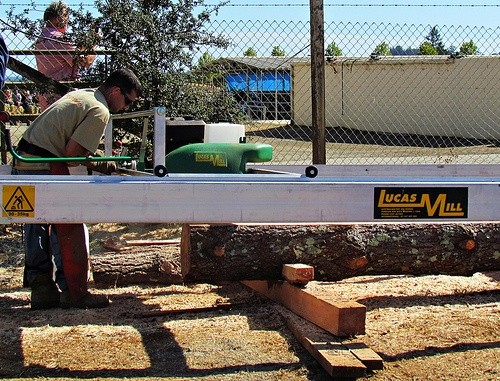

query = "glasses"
[117,86,133,106]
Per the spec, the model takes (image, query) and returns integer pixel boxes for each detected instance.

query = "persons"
[36,3,103,113]
[13,71,142,311]
[2,87,32,106]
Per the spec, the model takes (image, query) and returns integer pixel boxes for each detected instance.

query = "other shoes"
[31,291,64,308]
[64,292,108,308]
